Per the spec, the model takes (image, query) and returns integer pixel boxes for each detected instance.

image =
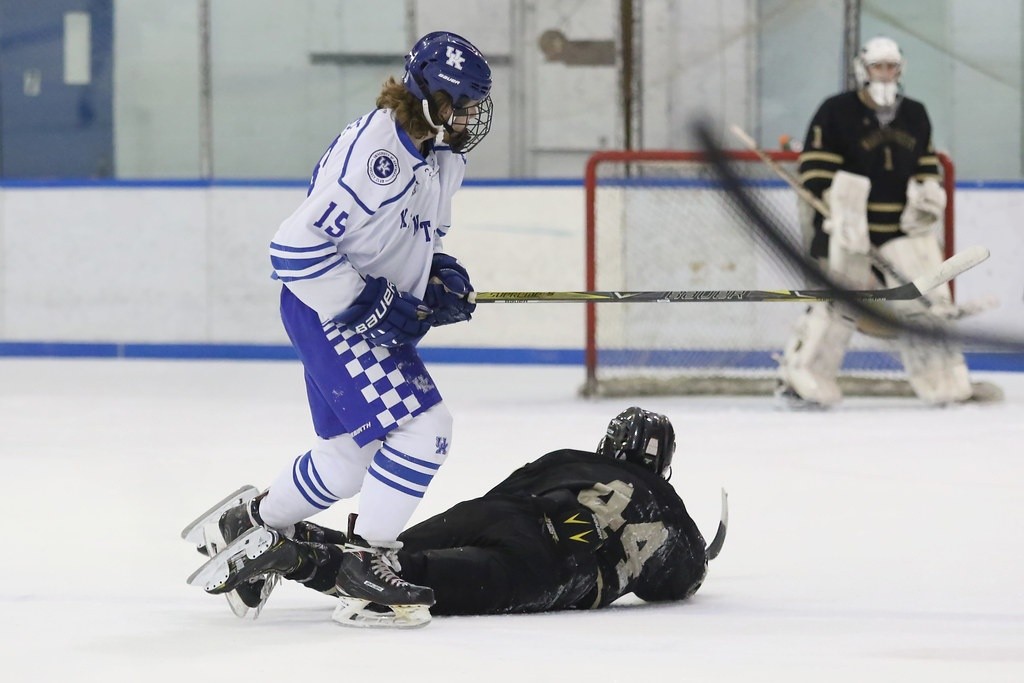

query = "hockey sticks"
[704,486,730,560]
[467,244,991,303]
[728,126,999,320]
[694,113,1020,352]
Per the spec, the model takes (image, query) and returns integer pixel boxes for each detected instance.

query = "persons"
[217,32,494,627]
[181,406,708,615]
[776,38,975,406]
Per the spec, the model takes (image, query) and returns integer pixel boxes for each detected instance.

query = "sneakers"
[187,525,346,595]
[179,484,260,557]
[328,513,436,626]
[219,486,269,621]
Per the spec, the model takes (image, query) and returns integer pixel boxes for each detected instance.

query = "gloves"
[422,253,477,327]
[330,274,435,350]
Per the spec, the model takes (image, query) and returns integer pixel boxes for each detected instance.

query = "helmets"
[853,37,903,111]
[401,31,494,154]
[595,405,676,481]
